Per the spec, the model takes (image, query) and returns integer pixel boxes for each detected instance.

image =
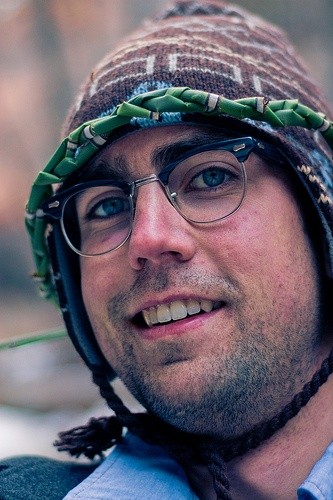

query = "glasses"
[41,136,273,260]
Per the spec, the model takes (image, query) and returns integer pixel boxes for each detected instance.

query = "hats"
[23,0,333,496]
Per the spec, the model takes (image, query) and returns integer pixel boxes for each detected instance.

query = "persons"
[0,0,333,500]
